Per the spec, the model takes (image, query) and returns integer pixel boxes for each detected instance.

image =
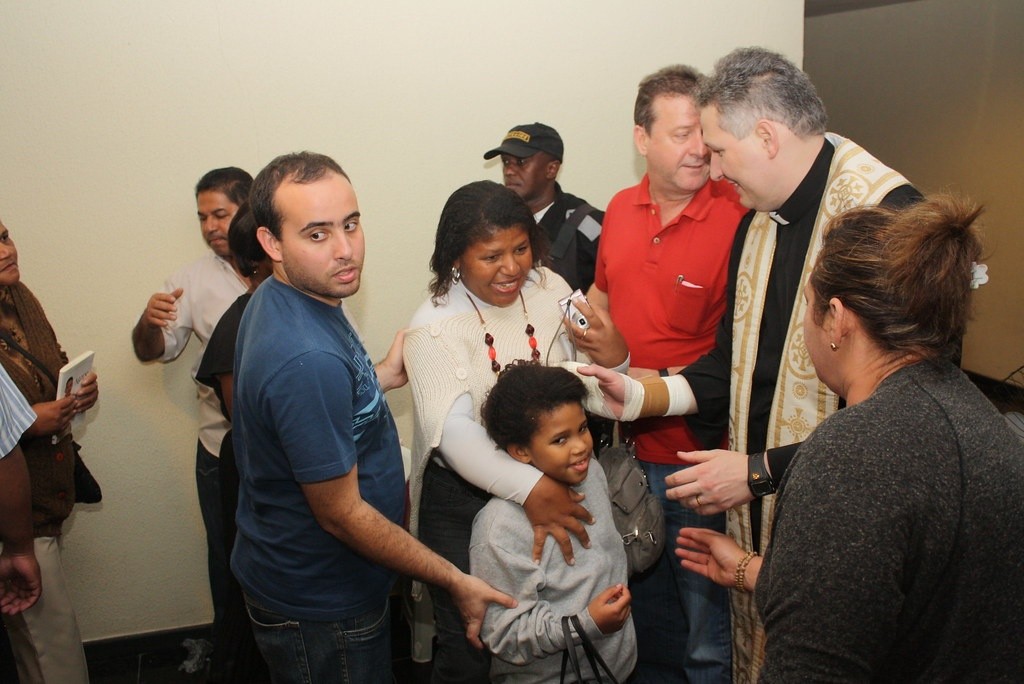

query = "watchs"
[748,451,776,497]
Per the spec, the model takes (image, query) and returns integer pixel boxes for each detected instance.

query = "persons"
[674,193,1024,684]
[468,365,639,684]
[559,48,964,684]
[58,376,77,441]
[229,149,518,684]
[482,122,615,459]
[131,162,273,683]
[586,64,751,684]
[403,180,597,684]
[0,221,103,684]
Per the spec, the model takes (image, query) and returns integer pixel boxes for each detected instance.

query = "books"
[51,351,95,444]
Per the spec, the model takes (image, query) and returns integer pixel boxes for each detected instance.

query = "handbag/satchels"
[72,442,102,504]
[596,437,664,576]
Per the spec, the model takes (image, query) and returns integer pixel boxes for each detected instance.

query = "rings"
[581,330,588,341]
[696,495,702,506]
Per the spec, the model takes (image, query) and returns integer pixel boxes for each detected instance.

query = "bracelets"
[735,550,759,590]
[658,368,669,377]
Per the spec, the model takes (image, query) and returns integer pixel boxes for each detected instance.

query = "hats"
[484,121,564,164]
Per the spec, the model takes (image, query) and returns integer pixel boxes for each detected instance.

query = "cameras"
[558,289,592,329]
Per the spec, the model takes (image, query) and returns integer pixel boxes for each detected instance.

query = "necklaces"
[463,289,541,375]
